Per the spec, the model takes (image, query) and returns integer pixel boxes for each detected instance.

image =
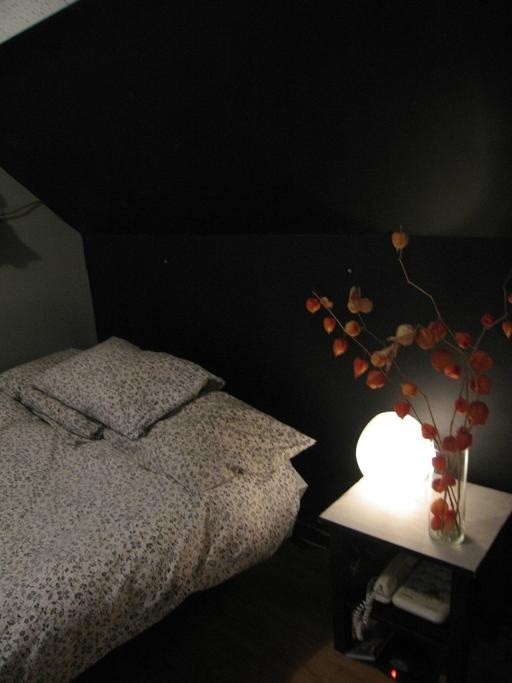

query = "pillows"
[0,346,108,438]
[33,338,225,438]
[104,392,312,498]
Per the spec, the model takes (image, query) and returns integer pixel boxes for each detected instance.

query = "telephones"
[371,549,452,625]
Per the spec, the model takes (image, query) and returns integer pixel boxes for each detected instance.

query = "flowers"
[305,225,512,536]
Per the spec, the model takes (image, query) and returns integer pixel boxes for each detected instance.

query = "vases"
[428,447,469,547]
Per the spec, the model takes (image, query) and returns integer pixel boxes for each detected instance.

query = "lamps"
[355,411,436,502]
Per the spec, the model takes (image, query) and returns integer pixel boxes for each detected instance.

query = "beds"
[0,338,315,683]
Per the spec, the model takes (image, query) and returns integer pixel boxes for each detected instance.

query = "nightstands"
[319,453,512,683]
